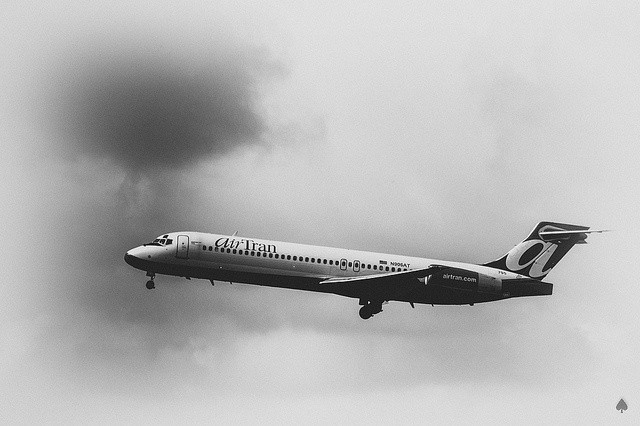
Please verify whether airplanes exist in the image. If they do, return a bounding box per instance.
[123,222,612,319]
